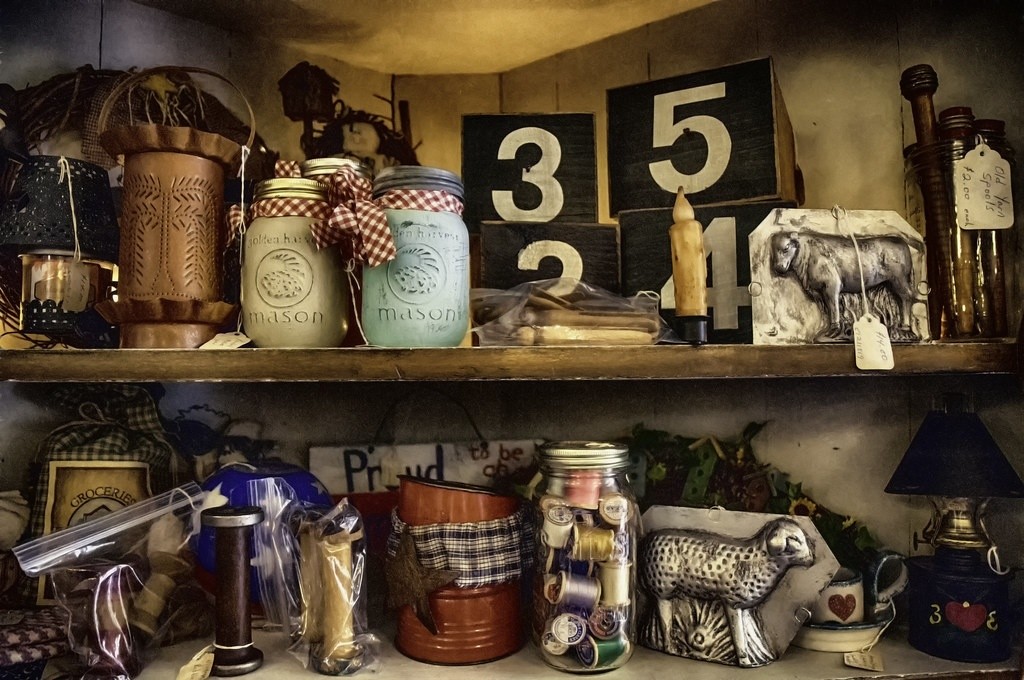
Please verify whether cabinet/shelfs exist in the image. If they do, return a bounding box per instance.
[0,339,1024,680]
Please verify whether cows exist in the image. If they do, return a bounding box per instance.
[769,227,919,339]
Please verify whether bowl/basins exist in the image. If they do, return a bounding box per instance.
[791,603,895,655]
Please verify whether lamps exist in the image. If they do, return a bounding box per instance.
[0,155,120,350]
[885,393,1023,663]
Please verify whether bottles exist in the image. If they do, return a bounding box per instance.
[357,165,472,348]
[297,156,372,348]
[238,178,354,349]
[531,438,640,674]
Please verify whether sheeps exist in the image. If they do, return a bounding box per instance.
[636,515,814,669]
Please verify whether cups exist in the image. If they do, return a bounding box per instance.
[811,568,865,627]
[904,135,1019,342]
[395,475,527,669]
